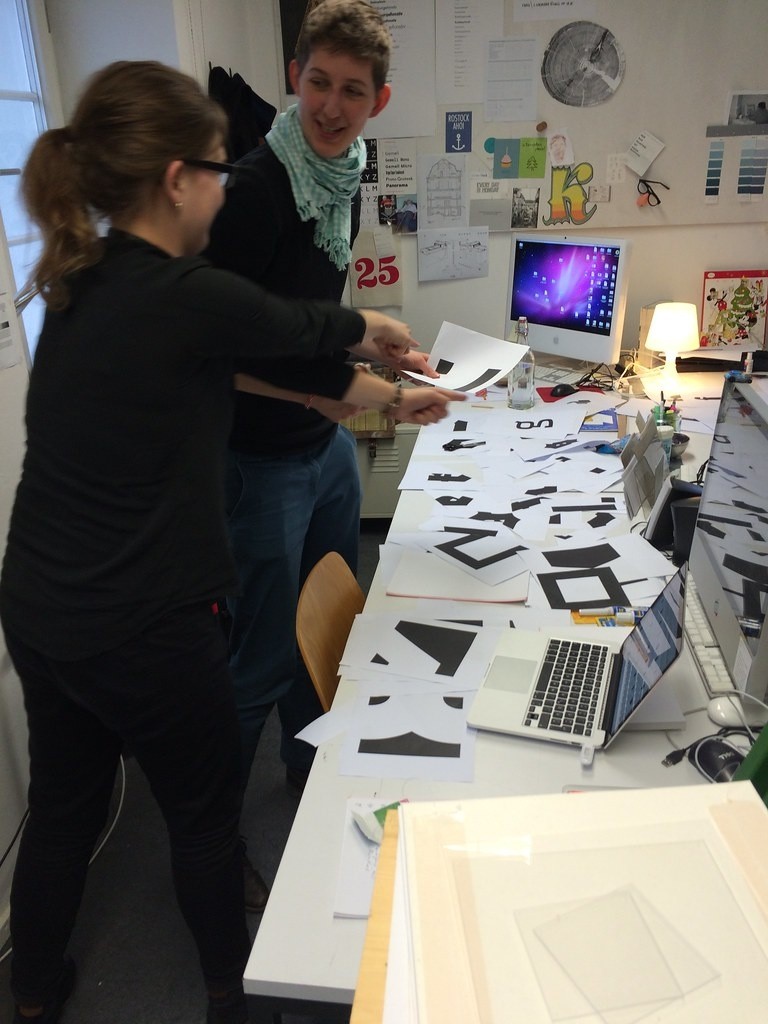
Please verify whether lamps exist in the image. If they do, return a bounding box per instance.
[645,301,700,391]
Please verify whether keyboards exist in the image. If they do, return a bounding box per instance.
[534,366,590,385]
[665,572,737,699]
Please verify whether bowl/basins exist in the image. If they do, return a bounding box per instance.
[671,432,690,457]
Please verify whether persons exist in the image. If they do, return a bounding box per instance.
[200,0,441,873]
[0,58,468,1024]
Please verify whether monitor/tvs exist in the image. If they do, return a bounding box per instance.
[505,232,631,375]
[687,375,768,702]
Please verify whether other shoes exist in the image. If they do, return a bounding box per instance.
[284,767,308,797]
[241,833,269,911]
[207,989,249,1023]
[14,953,76,1023]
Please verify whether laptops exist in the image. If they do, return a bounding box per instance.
[467,561,689,752]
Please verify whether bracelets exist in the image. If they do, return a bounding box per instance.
[304,392,317,410]
[381,384,403,419]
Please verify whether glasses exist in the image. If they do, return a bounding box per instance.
[163,153,244,191]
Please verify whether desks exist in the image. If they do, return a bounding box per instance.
[240,357,768,1009]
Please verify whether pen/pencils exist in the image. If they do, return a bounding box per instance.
[472,405,493,408]
[659,391,665,426]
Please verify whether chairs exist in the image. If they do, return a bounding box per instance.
[295,551,367,713]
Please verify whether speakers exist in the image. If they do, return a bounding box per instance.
[643,476,702,551]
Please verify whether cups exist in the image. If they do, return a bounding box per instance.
[656,426,674,467]
[647,408,680,432]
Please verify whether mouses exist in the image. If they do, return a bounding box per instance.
[708,696,768,728]
[550,384,579,397]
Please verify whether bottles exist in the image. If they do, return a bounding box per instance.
[744,352,754,372]
[507,316,535,410]
